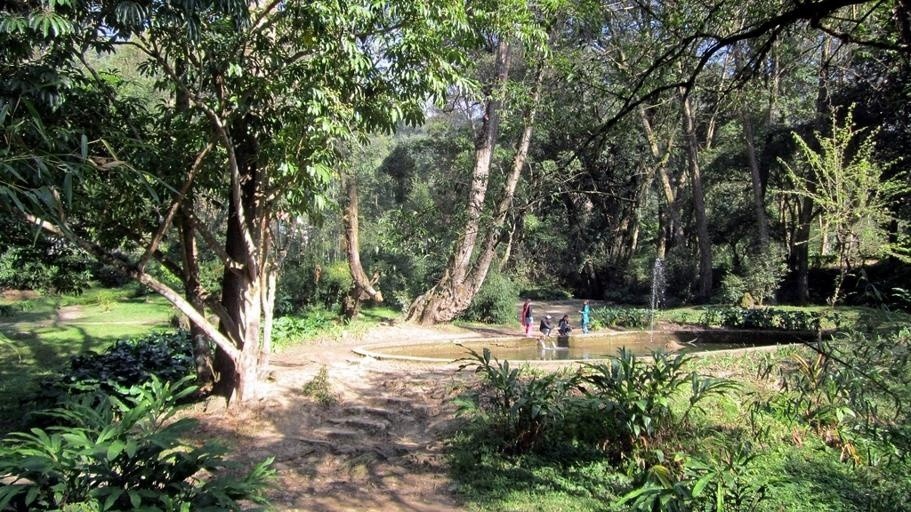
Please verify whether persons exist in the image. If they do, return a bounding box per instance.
[523,299,533,337]
[578,300,590,333]
[558,315,570,336]
[540,315,554,336]
